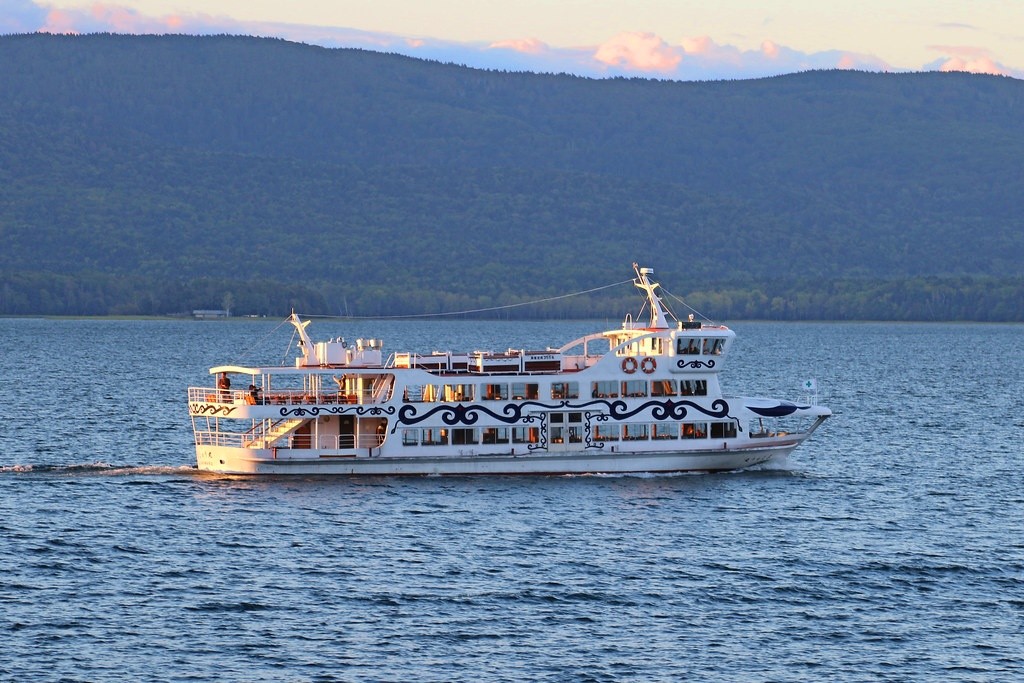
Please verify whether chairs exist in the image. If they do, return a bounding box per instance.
[205,393,348,404]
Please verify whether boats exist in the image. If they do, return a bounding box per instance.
[187,261,831,478]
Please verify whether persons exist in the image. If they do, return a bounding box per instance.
[248,385,271,405]
[678,339,694,354]
[703,339,708,351]
[218,372,232,402]
[376,418,387,446]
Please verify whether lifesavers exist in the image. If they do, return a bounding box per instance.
[641,357,657,374]
[621,357,638,374]
[342,341,347,348]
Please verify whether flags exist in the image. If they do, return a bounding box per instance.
[802,379,817,390]
